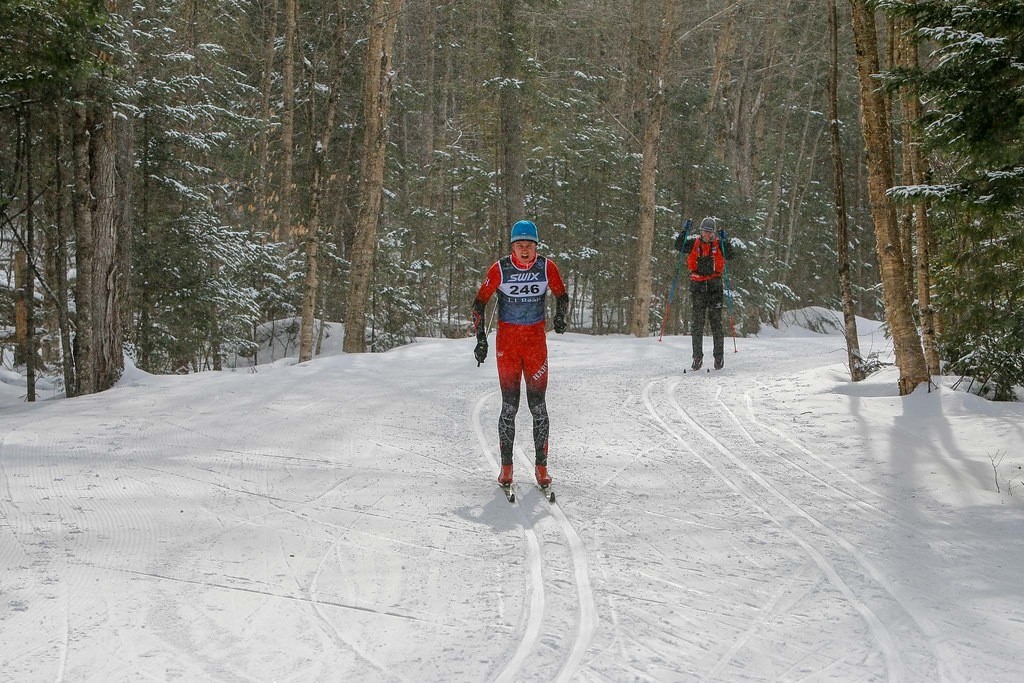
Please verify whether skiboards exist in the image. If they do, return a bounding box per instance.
[683,364,716,374]
[502,482,556,503]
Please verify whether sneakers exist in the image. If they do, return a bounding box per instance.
[498,464,513,485]
[692,357,703,370]
[714,355,724,369]
[534,465,552,485]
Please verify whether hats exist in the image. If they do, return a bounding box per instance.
[510,220,538,245]
[699,218,716,233]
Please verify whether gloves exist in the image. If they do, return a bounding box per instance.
[718,229,727,239]
[553,316,567,334]
[474,334,488,363]
[683,220,692,230]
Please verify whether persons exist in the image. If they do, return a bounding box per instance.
[675,217,736,371]
[472,220,570,485]
